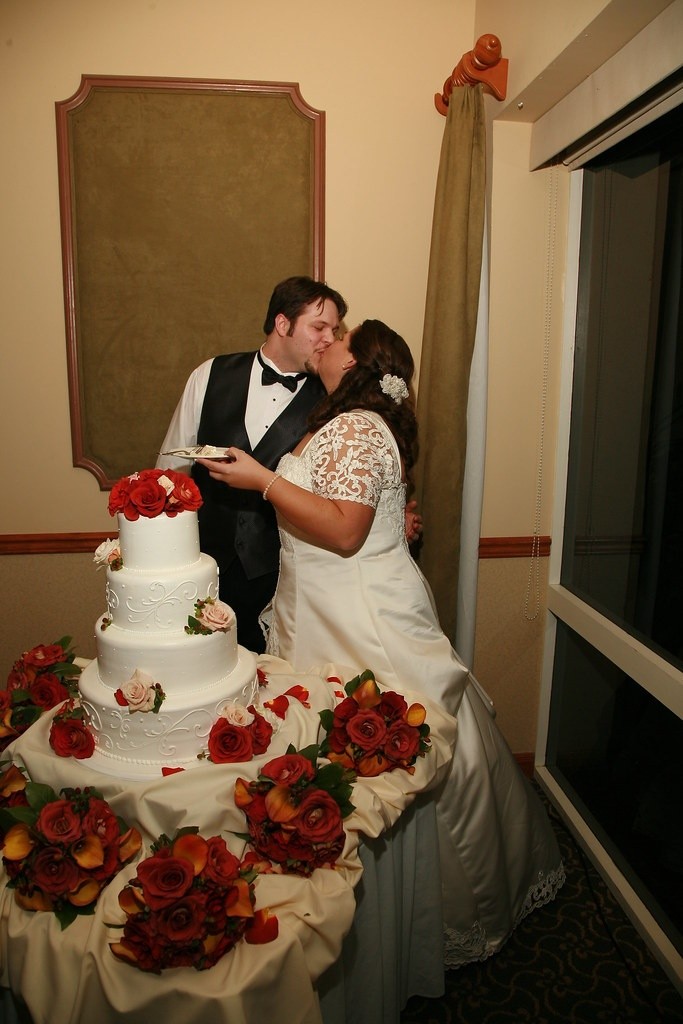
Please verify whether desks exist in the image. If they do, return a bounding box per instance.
[0,656,460,1024]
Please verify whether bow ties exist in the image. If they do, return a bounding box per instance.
[257,350,308,392]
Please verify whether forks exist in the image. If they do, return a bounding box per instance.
[154,443,203,456]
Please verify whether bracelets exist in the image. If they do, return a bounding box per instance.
[263,473,281,499]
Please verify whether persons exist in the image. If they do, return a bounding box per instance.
[151,275,423,654]
[195,319,566,968]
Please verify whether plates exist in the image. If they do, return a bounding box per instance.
[170,446,244,461]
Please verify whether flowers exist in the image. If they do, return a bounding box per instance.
[225,742,357,877]
[378,374,409,406]
[317,668,434,777]
[107,467,204,521]
[93,537,121,571]
[119,668,167,713]
[102,825,258,976]
[208,701,273,763]
[1,760,143,931]
[0,634,95,759]
[183,596,237,636]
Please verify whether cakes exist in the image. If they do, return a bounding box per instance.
[49,468,274,766]
